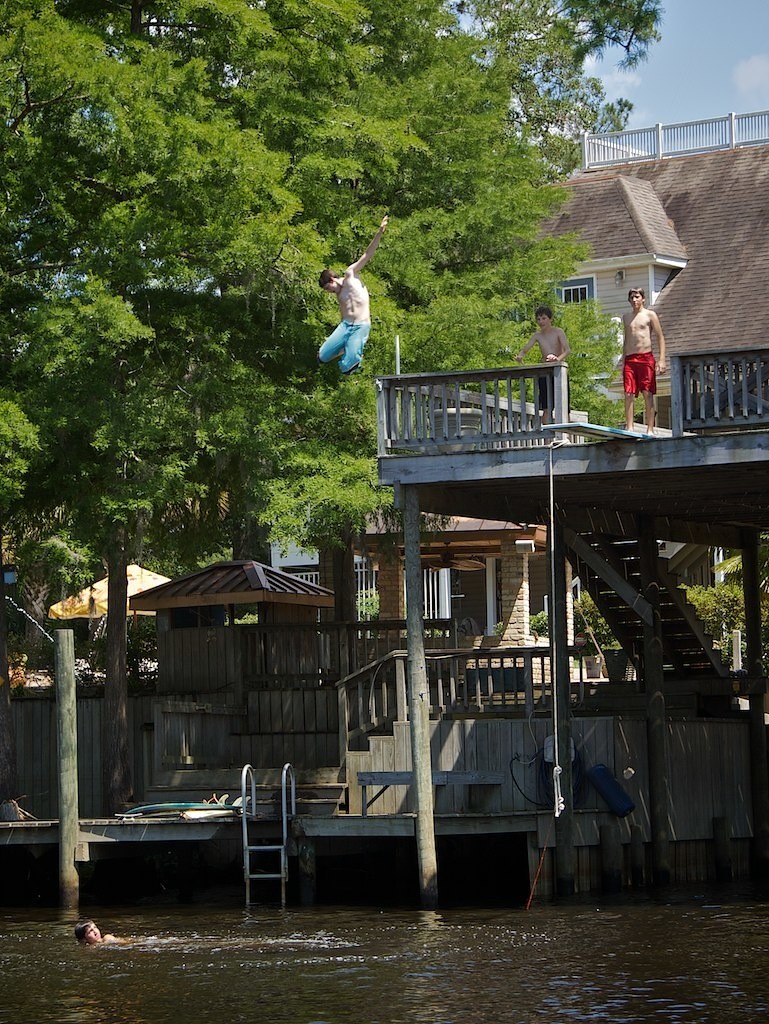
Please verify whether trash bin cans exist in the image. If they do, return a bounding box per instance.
[584,656,601,678]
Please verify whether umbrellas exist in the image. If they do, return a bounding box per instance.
[48,562,172,629]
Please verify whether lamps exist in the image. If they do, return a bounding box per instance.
[614,269,625,288]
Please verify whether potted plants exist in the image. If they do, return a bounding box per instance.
[573,591,628,681]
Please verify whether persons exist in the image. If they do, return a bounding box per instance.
[75,920,115,944]
[514,307,571,444]
[623,288,665,436]
[318,215,390,375]
